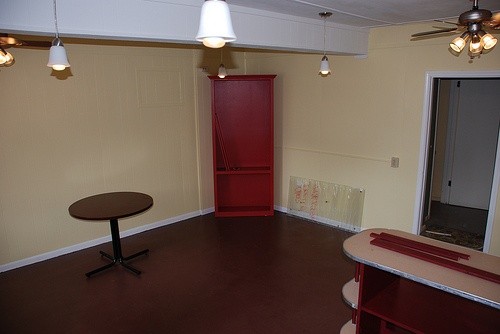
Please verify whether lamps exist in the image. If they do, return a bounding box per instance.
[449,24,498,53]
[47,0,70,70]
[0,33,19,65]
[196,0,237,48]
[217,47,228,78]
[318,12,334,75]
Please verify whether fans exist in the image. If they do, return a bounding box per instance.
[411,0,499,38]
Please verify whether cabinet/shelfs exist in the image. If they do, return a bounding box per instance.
[338,229,500,334]
[208,74,278,216]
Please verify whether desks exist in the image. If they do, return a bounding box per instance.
[69,191,153,279]
